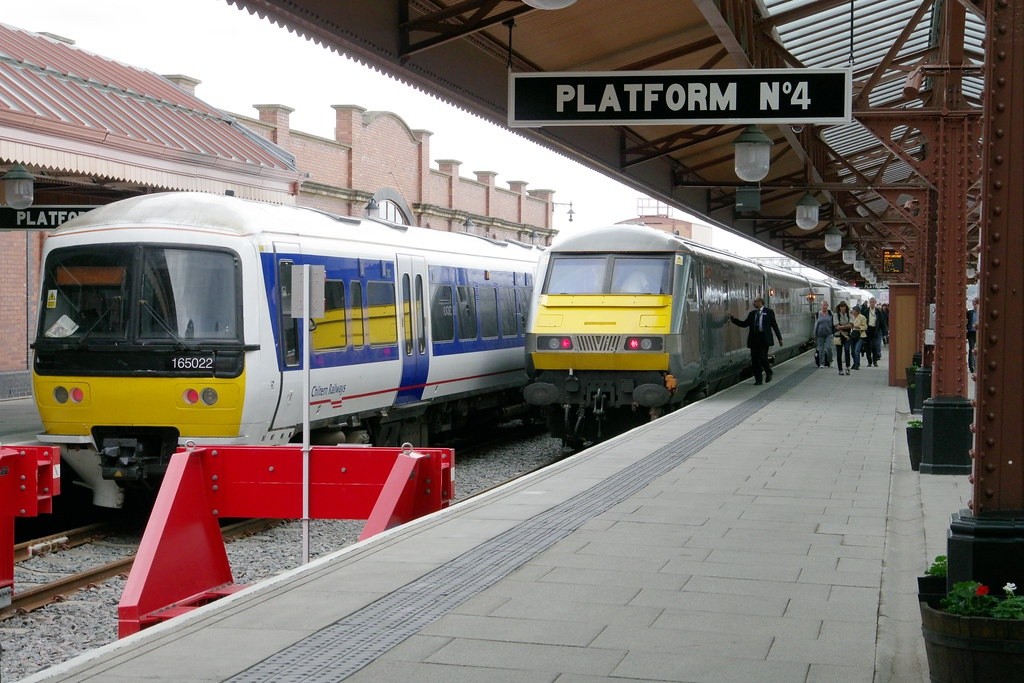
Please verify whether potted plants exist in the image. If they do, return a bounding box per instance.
[907,420,923,472]
[906,366,919,413]
[918,556,948,595]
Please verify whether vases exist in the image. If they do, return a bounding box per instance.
[920,600,1024,683]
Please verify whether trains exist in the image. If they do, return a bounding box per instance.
[523,222,869,449]
[30,191,548,521]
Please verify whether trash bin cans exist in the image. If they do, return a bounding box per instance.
[913,352,922,367]
[919,394,974,475]
[914,367,932,412]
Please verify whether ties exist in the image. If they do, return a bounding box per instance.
[754,311,761,337]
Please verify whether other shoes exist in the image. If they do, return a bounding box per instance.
[851,364,859,370]
[765,371,773,383]
[867,363,871,367]
[839,371,844,375]
[820,365,824,369]
[845,369,850,375]
[873,362,878,367]
[754,382,762,385]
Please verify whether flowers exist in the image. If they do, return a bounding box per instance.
[941,581,1024,620]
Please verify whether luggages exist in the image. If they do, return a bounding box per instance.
[814,339,830,368]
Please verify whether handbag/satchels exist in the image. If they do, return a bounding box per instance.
[851,330,860,343]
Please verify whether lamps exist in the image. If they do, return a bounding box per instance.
[365,198,379,217]
[853,254,877,284]
[824,222,843,254]
[732,125,774,182]
[0,164,36,210]
[903,66,926,99]
[528,230,539,245]
[840,243,857,264]
[904,200,919,213]
[794,190,821,230]
[552,202,575,222]
[460,217,474,235]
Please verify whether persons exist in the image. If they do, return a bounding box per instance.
[966,298,978,372]
[730,297,783,385]
[815,297,889,375]
[706,311,731,357]
